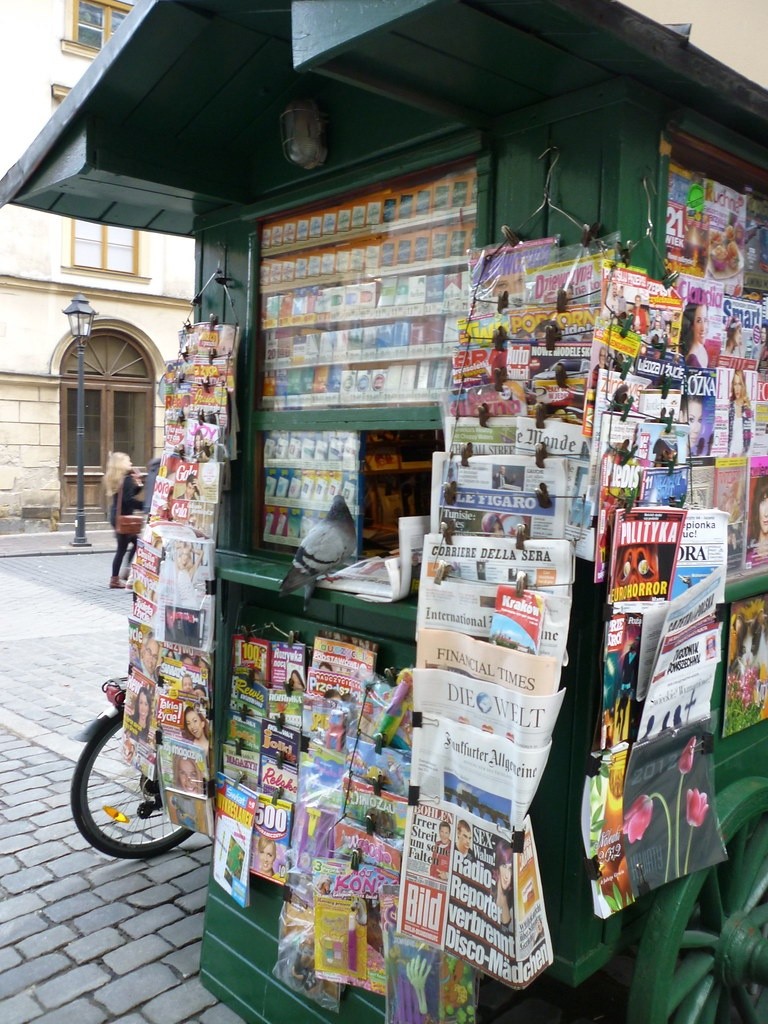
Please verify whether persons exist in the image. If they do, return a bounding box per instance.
[145,458,161,524]
[481,295,768,569]
[129,475,513,924]
[101,452,144,588]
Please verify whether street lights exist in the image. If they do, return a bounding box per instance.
[61,293,99,548]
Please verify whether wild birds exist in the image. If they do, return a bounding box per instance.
[278,495,356,611]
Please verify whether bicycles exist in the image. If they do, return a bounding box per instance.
[69,678,195,860]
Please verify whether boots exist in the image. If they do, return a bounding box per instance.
[110,577,126,588]
[124,569,132,580]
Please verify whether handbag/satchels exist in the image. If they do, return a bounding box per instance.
[116,515,144,534]
